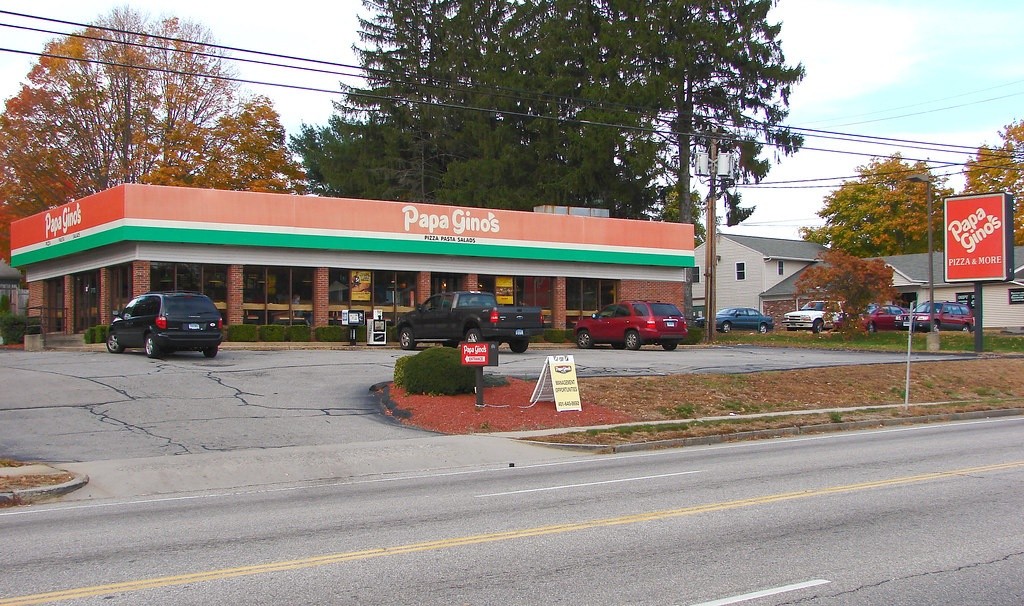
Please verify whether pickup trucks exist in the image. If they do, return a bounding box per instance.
[395,289,544,353]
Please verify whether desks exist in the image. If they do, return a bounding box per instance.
[274,316,305,321]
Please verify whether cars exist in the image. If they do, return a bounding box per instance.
[833,304,909,332]
[694,307,775,334]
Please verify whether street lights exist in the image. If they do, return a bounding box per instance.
[906,172,940,354]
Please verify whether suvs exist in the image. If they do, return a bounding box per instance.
[573,299,689,351]
[894,299,977,332]
[782,300,847,332]
[106,289,224,359]
